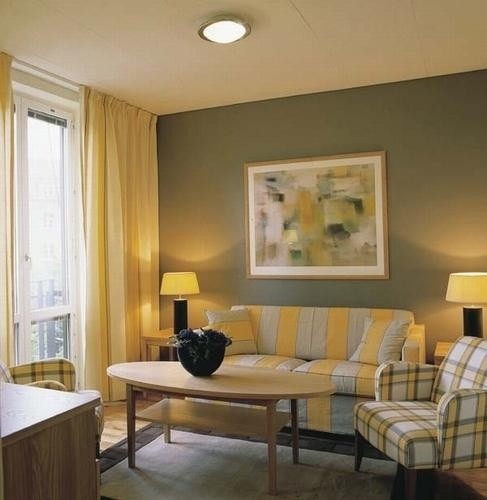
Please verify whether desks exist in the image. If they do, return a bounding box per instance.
[139,326,179,400]
[431,341,457,367]
[105,359,337,495]
[0,379,106,500]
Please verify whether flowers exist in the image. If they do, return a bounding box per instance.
[162,324,234,366]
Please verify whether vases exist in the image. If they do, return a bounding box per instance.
[175,340,228,378]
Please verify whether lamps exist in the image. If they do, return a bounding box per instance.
[194,10,252,45]
[156,268,201,336]
[443,271,486,338]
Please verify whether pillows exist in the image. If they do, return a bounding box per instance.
[347,315,413,368]
[201,304,259,357]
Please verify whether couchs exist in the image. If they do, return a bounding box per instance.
[180,302,428,446]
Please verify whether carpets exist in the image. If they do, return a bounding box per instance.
[95,418,413,500]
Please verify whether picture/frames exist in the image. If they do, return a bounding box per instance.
[241,148,393,283]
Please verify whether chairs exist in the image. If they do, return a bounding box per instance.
[0,355,105,462]
[351,332,486,500]
